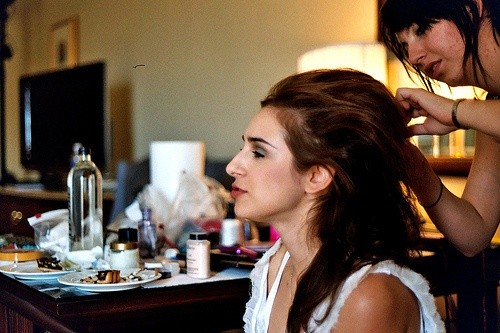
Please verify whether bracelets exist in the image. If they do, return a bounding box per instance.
[418,175,443,208]
[452,98,470,130]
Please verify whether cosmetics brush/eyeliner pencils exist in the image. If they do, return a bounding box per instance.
[164,248,219,264]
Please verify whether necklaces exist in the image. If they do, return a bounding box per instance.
[289,264,294,301]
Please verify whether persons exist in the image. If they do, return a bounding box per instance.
[378,0,500,258]
[226,67,446,333]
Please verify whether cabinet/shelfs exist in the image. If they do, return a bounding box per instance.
[0,183,121,238]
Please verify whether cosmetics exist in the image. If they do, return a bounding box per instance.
[185,232,211,278]
[110,228,140,270]
[222,203,258,258]
[67,146,105,252]
[138,208,156,259]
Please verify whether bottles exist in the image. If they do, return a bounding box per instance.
[138,208,157,262]
[186,232,211,279]
[220,201,243,246]
[66,147,102,253]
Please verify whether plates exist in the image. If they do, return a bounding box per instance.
[210,246,273,268]
[0,259,163,293]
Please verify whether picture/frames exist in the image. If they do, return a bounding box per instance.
[47,15,80,70]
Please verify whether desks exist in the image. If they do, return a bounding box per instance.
[0,234,436,332]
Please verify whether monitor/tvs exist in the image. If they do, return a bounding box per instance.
[19,60,111,190]
